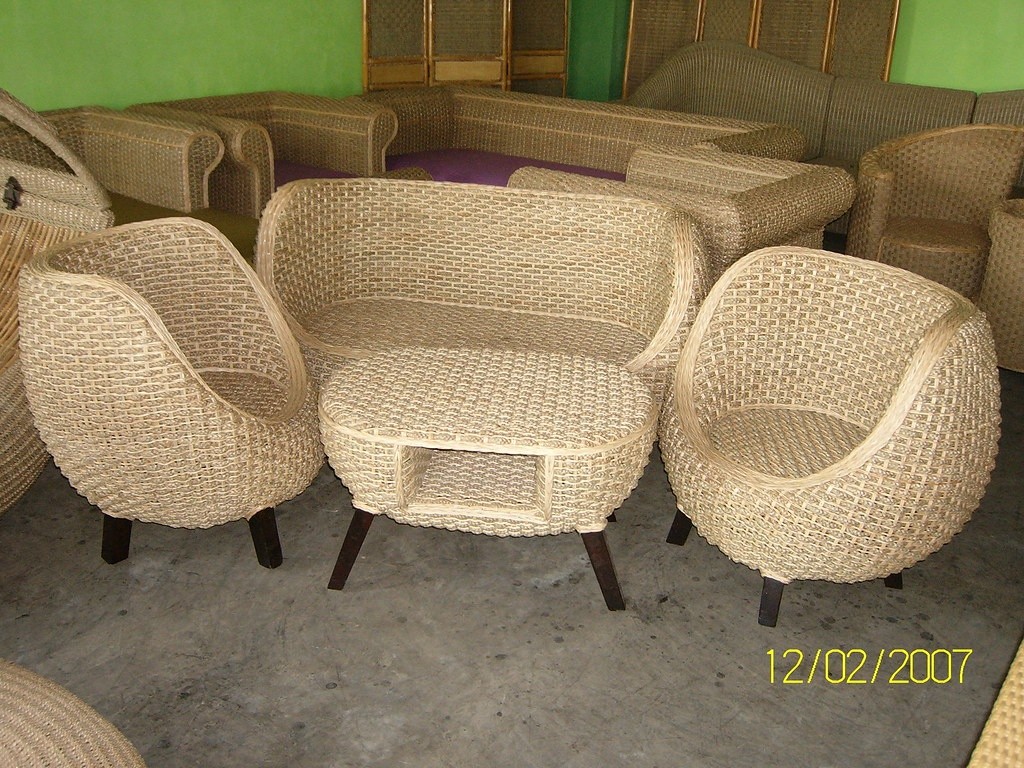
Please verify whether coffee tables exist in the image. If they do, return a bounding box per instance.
[317,349,657,612]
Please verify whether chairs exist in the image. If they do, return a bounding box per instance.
[656,244,1003,628]
[845,123,1024,294]
[126,91,400,220]
[0,105,258,258]
[19,217,324,568]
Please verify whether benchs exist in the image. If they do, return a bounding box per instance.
[625,41,1024,237]
[345,85,804,188]
[258,177,712,523]
